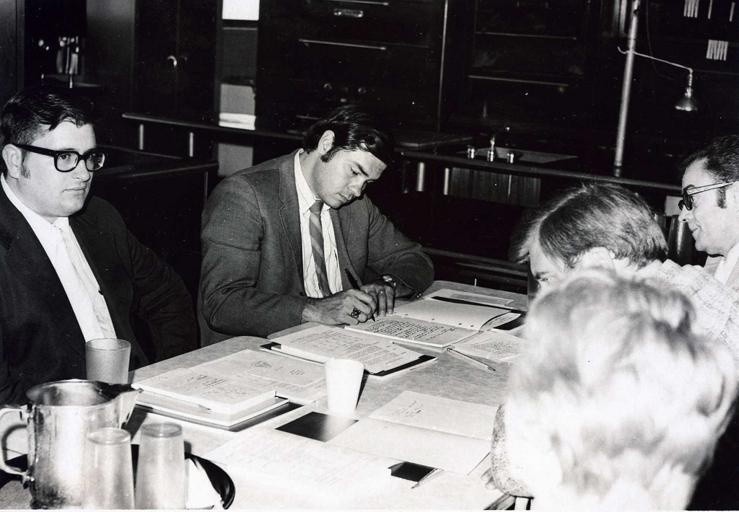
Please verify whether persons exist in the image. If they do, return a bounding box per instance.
[0,87,196,407]
[197,104,436,347]
[492,180,738,499]
[504,266,738,510]
[677,137,739,289]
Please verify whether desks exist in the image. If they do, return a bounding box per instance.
[1,280,530,510]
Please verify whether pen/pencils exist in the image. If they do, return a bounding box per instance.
[344,268,375,323]
[447,348,496,372]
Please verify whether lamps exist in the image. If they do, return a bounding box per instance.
[617,46,699,111]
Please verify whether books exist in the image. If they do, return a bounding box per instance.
[132,294,527,477]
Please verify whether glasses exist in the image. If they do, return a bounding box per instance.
[14,143,106,172]
[679,182,733,210]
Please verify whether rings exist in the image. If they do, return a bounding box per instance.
[351,308,361,319]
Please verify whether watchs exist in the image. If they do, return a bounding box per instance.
[379,275,398,289]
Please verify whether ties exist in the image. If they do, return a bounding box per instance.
[309,200,333,298]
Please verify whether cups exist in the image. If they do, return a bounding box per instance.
[0,337,185,509]
[325,358,365,414]
